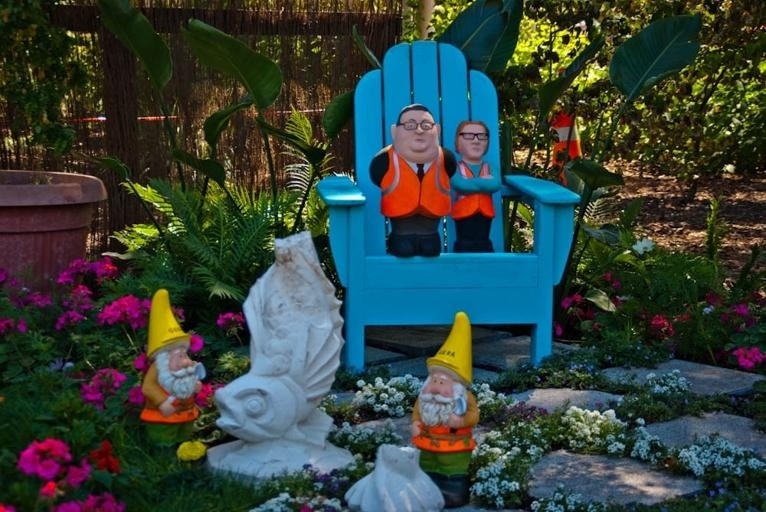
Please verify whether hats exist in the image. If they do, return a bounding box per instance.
[145,287,192,358]
[425,310,475,385]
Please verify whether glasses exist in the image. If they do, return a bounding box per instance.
[397,121,437,131]
[457,132,488,142]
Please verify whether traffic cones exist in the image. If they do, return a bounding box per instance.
[553,67,583,186]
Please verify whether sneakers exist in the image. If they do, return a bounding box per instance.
[440,475,472,504]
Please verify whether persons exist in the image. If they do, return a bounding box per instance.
[453,120,495,253]
[408,311,480,506]
[371,102,455,259]
[142,287,205,452]
